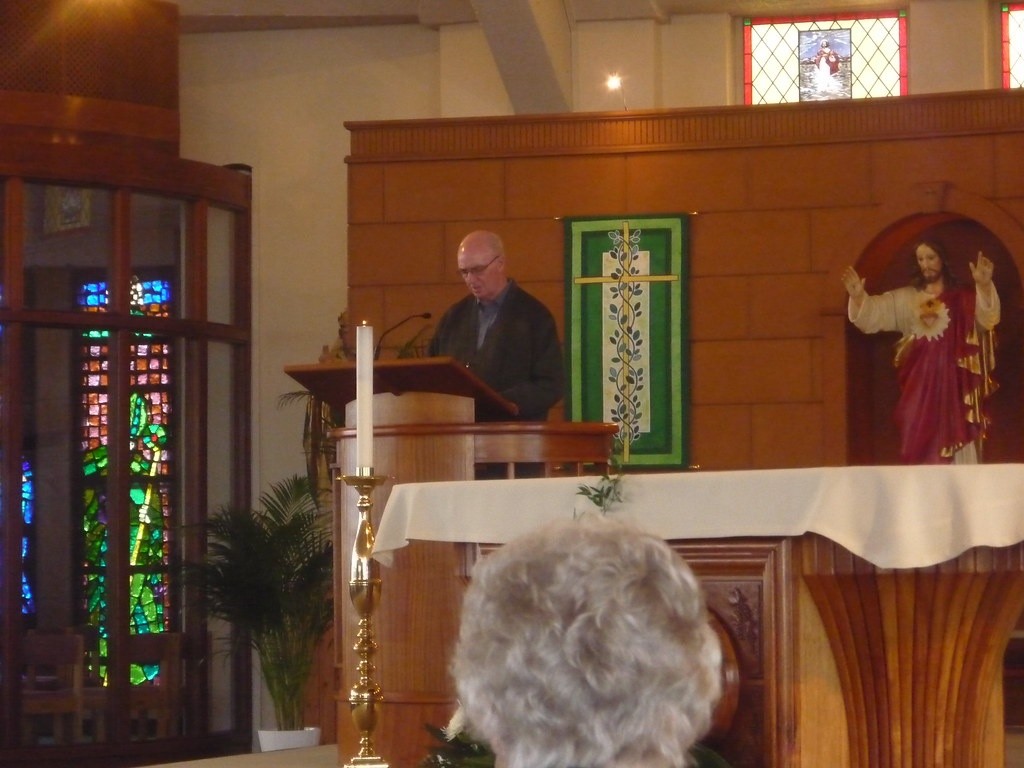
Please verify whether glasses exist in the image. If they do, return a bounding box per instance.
[456,256,498,275]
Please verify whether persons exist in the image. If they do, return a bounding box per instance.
[839,238,1003,465]
[445,510,724,767]
[425,229,565,483]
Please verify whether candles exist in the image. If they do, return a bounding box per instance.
[356,319,373,475]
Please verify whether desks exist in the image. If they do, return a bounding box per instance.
[370,464,1024,766]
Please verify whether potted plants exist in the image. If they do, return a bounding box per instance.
[180,474,342,754]
